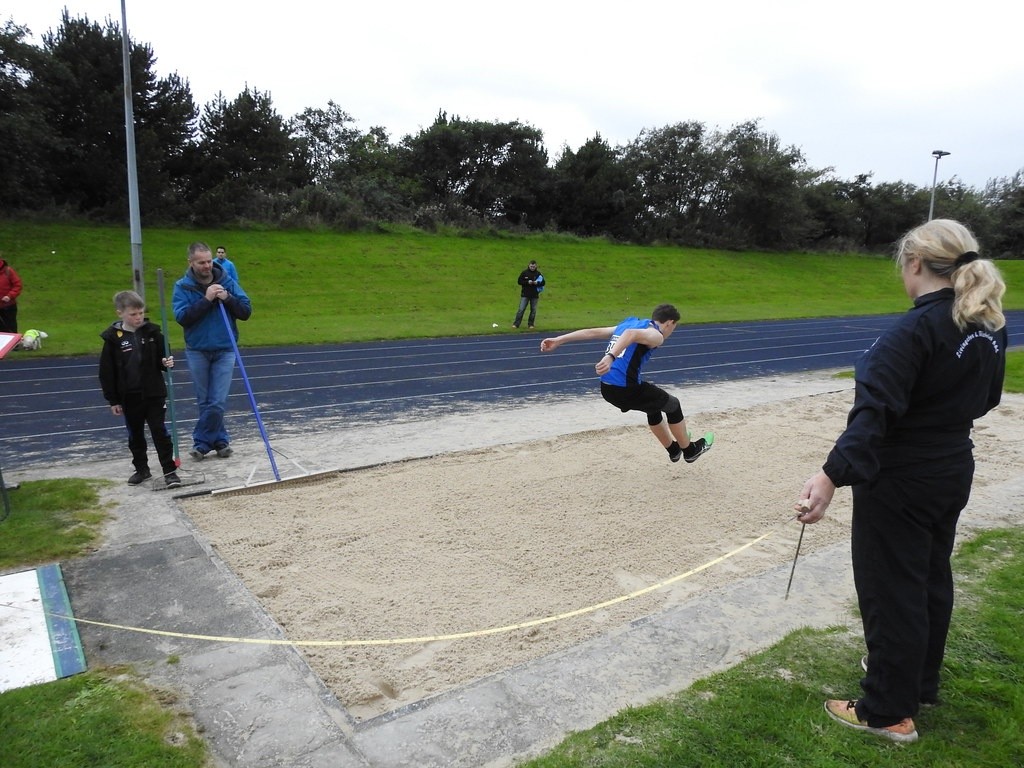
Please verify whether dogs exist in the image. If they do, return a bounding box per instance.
[20,329,49,351]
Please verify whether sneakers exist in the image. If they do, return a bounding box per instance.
[217,445,234,457]
[163,471,181,488]
[669,432,692,462]
[188,449,203,462]
[684,432,714,463]
[824,698,919,744]
[860,654,937,708]
[127,466,153,485]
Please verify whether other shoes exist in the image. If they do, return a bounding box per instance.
[528,326,534,329]
[512,325,517,329]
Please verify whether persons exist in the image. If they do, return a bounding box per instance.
[512,260,545,329]
[172,241,252,460]
[541,304,713,464]
[99,290,181,488]
[795,218,1009,740]
[0,258,22,351]
[212,246,239,343]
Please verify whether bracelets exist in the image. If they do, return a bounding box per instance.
[607,353,615,362]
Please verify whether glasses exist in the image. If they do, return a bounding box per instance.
[217,252,224,254]
